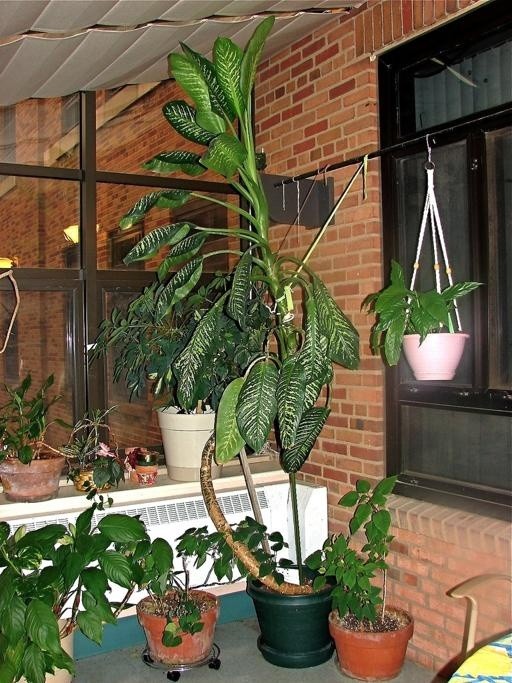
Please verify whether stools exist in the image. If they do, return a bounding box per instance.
[431,573,512,682]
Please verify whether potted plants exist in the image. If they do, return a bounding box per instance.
[88,16,359,669]
[2,368,161,500]
[360,254,483,385]
[302,472,417,683]
[2,504,168,683]
